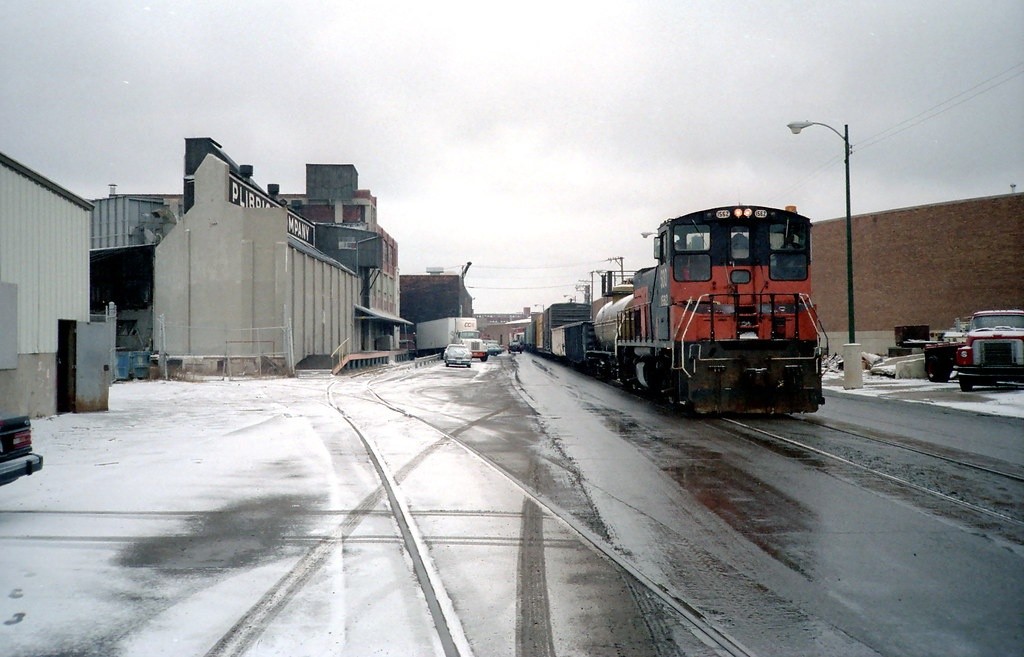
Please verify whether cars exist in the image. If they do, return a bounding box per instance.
[483,340,506,356]
[508,340,522,354]
[447,347,472,368]
[444,344,465,363]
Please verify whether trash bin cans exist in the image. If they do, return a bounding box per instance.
[133,351,150,378]
[115,351,131,379]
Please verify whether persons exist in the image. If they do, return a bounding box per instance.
[780,234,801,249]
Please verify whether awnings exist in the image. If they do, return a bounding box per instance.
[354,305,414,326]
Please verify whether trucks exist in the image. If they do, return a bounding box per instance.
[416,317,489,362]
[889,309,1024,392]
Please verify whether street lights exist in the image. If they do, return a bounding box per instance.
[535,303,544,311]
[563,294,577,302]
[787,120,863,390]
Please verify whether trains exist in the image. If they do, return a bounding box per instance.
[509,205,830,419]
